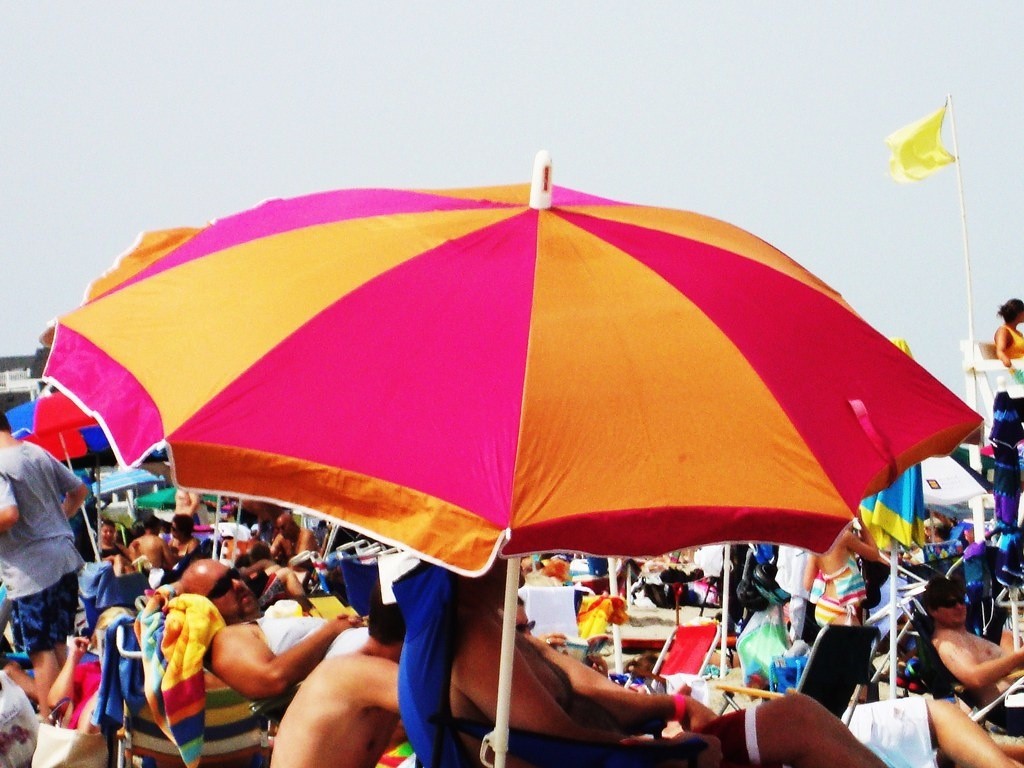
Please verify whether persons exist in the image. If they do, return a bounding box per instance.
[0,298,1024,768]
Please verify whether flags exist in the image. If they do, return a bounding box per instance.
[883,99,956,185]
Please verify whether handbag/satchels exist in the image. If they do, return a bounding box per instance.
[1,670,111,767]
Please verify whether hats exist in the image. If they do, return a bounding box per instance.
[923,517,943,528]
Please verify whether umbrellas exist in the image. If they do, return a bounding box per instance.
[4,149,985,768]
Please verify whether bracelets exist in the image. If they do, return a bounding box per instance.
[668,693,686,723]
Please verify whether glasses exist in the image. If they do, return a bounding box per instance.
[937,596,965,608]
[207,568,241,598]
[516,620,536,634]
[275,525,287,531]
[171,526,178,533]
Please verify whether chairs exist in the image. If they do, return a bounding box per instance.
[81,539,1024,768]
